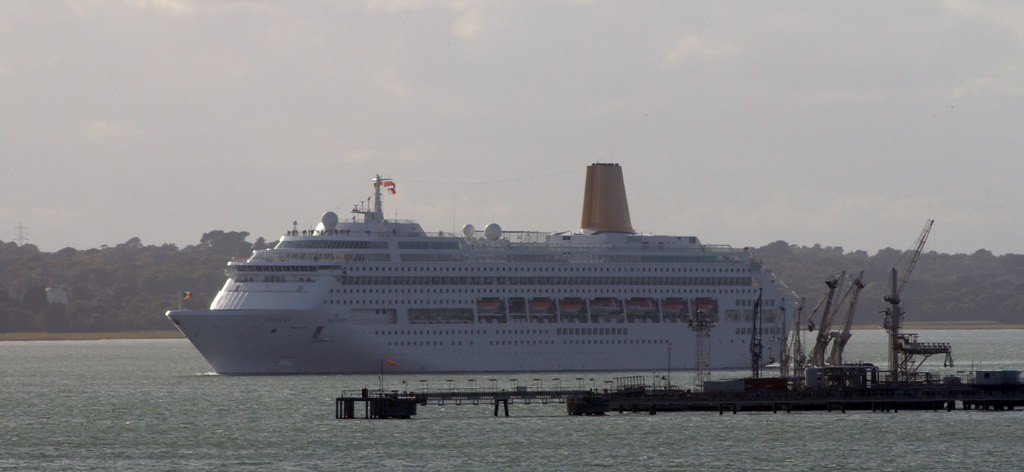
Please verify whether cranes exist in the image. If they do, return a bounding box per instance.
[882,217,957,374]
[748,266,867,388]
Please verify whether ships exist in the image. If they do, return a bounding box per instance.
[166,160,794,375]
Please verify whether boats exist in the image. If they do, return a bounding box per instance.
[588,298,622,316]
[478,300,502,311]
[557,301,584,313]
[661,300,689,314]
[529,300,553,312]
[693,300,716,314]
[625,297,658,315]
